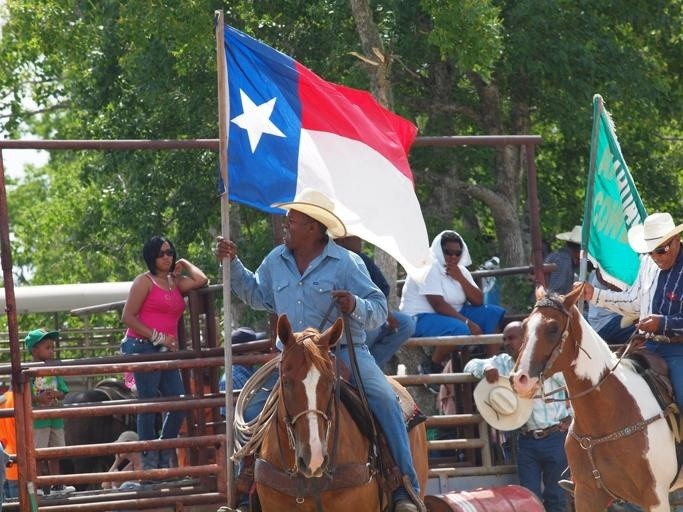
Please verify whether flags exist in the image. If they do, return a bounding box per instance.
[221,18,432,287]
[584,92,646,295]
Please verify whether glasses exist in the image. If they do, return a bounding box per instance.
[647,237,675,254]
[155,248,175,257]
[441,248,462,257]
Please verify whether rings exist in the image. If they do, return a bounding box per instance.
[171,341,175,346]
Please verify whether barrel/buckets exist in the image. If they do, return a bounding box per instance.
[424,483,543,511]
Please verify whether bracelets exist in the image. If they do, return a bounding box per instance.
[148,327,165,347]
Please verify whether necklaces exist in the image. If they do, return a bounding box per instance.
[154,271,172,291]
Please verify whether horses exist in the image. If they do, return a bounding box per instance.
[250,311,430,512]
[506,278,682,512]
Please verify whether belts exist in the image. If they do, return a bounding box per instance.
[645,334,683,344]
[514,424,563,440]
[328,343,363,350]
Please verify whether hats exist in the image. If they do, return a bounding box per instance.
[230,327,269,344]
[626,212,683,254]
[472,375,534,432]
[554,225,583,244]
[270,189,347,238]
[24,327,59,353]
[113,430,140,443]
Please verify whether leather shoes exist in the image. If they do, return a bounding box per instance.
[217,505,248,512]
[558,479,575,494]
[395,499,417,512]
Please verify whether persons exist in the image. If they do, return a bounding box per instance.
[331,235,416,372]
[462,319,576,511]
[215,190,426,511]
[116,232,209,485]
[99,429,140,489]
[397,228,507,397]
[0,373,18,497]
[217,325,270,418]
[23,325,76,498]
[580,211,682,417]
[531,221,582,298]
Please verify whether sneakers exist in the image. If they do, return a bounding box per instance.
[141,476,183,490]
[35,483,77,498]
[417,359,443,395]
